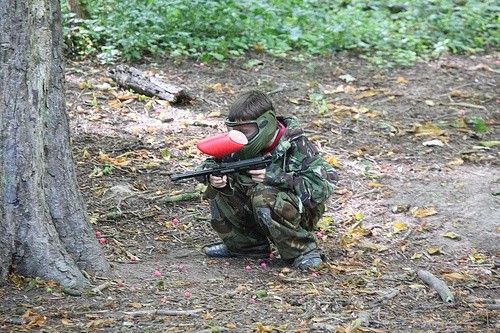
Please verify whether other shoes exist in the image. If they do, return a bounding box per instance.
[201,240,271,259]
[295,251,323,270]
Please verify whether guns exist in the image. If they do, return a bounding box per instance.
[170,130,272,189]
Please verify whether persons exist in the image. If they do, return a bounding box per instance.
[195,91,339,273]
